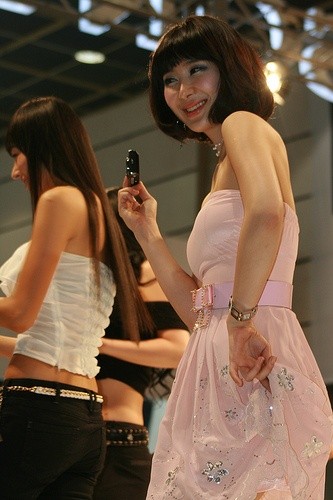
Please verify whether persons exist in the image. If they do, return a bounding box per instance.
[0,186,194,500]
[0,97,154,500]
[117,17,333,500]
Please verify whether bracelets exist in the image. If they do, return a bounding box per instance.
[228,294,259,321]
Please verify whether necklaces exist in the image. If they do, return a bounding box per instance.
[212,136,225,156]
